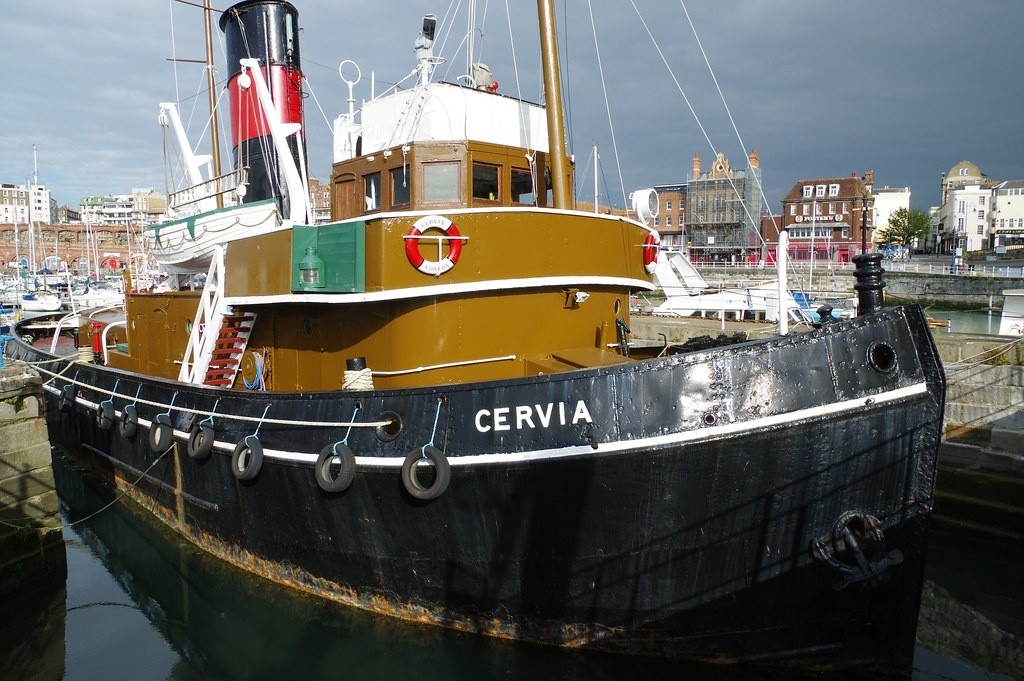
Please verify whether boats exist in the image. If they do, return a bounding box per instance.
[0,0,1023,681]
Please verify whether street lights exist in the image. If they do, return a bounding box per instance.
[860,194,868,254]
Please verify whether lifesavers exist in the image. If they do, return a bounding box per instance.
[642,228,661,276]
[405,218,461,276]
